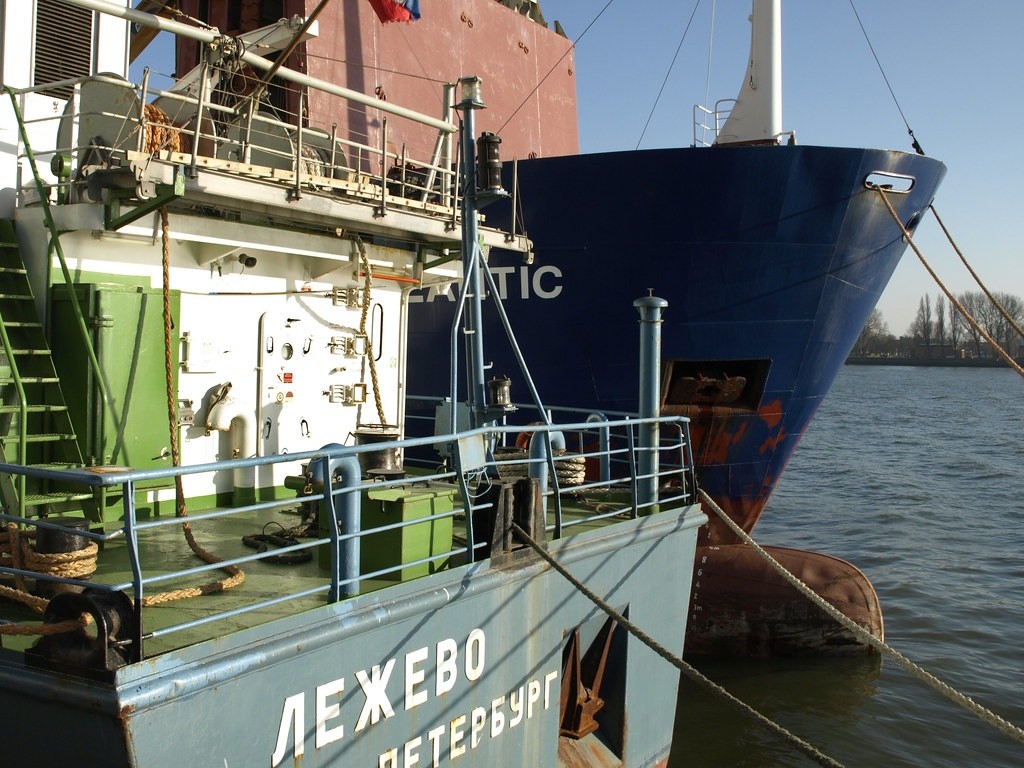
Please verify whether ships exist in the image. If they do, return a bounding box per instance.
[0,1,952,768]
